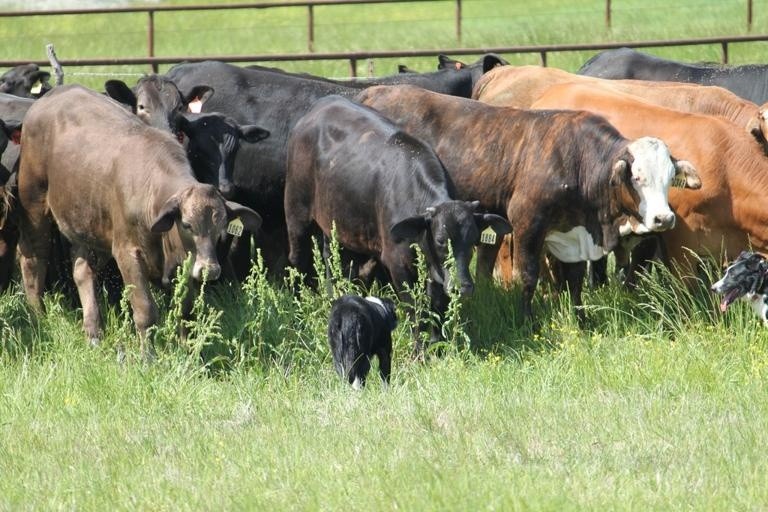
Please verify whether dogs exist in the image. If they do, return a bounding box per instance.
[327,293,398,394]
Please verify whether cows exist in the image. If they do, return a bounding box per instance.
[0,46,768,370]
